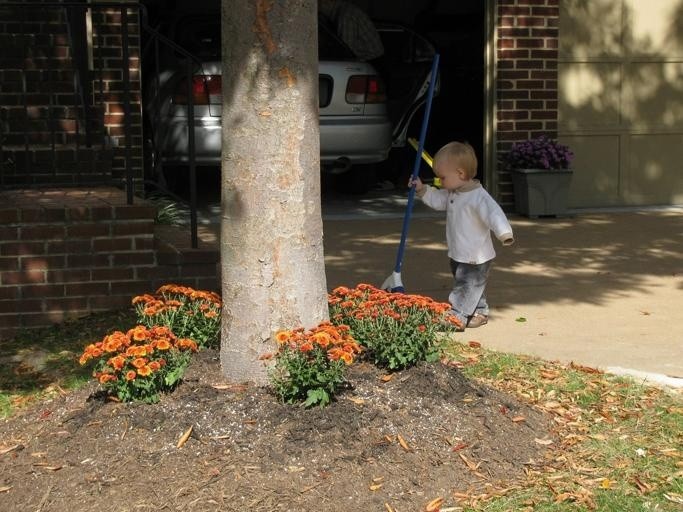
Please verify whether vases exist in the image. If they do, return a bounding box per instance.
[510,165,575,219]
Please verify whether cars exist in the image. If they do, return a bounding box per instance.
[141,13,392,196]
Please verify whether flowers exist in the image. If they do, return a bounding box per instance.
[505,134,575,170]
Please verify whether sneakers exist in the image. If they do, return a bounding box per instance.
[437,317,464,332]
[466,315,489,328]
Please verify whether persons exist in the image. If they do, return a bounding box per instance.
[408,143,516,330]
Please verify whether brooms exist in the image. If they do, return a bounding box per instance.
[378,52,440,295]
